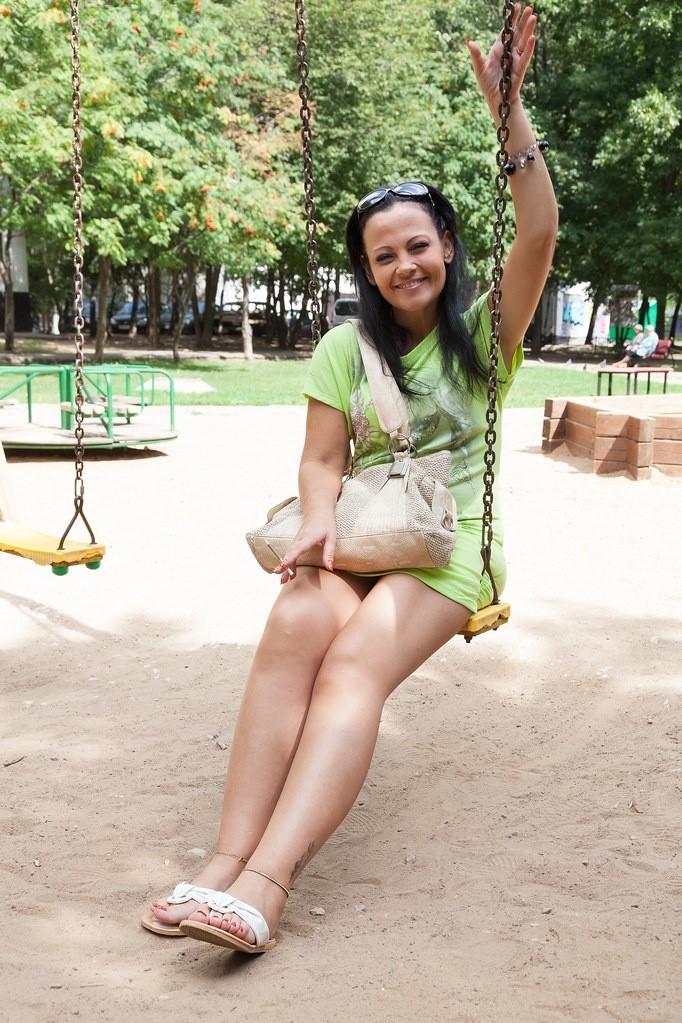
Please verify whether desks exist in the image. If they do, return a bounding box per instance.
[598,367,670,395]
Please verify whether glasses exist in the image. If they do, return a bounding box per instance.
[357,181,436,220]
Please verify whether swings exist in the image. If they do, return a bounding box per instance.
[294,0,516,642]
[0,0,109,579]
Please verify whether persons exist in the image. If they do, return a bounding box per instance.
[140,1,558,953]
[612,324,659,368]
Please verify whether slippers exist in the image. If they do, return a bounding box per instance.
[141,881,224,936]
[179,892,276,954]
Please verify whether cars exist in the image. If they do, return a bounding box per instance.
[110,301,182,333]
[160,301,222,335]
[258,310,328,336]
[58,300,123,334]
[331,299,362,328]
[219,301,288,337]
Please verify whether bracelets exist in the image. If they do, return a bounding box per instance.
[502,140,549,177]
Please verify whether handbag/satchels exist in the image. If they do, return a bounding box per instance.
[245,319,457,574]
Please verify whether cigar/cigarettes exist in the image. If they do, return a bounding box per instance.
[264,540,294,576]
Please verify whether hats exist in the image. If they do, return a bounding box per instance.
[646,326,654,332]
[634,324,643,330]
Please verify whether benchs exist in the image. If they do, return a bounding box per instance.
[618,339,675,367]
